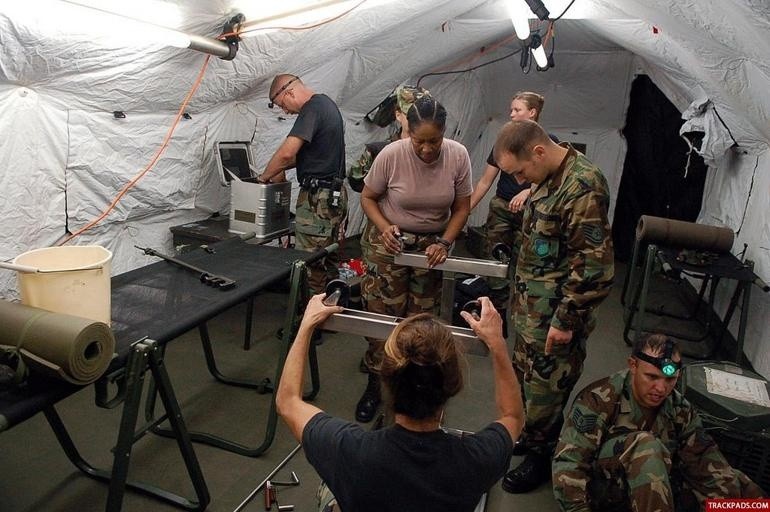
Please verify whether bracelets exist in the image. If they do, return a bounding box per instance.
[256,172,266,184]
[434,236,452,250]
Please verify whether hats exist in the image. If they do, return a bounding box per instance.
[398,85,433,114]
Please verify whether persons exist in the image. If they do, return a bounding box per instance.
[548,332,770,510]
[355,95,476,423]
[490,113,617,495]
[466,89,559,339]
[255,72,348,349]
[348,82,433,374]
[274,288,530,511]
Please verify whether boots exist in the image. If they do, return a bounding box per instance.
[499,435,552,494]
[356,373,381,424]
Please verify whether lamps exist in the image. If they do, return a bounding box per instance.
[169,15,246,60]
[528,35,550,71]
[506,0,531,41]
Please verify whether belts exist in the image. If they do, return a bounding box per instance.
[401,231,426,246]
[318,181,331,190]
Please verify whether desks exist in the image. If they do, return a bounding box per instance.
[0,239,339,512]
[620,236,770,370]
[169,215,298,349]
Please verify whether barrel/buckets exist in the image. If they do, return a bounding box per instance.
[0,243,114,327]
